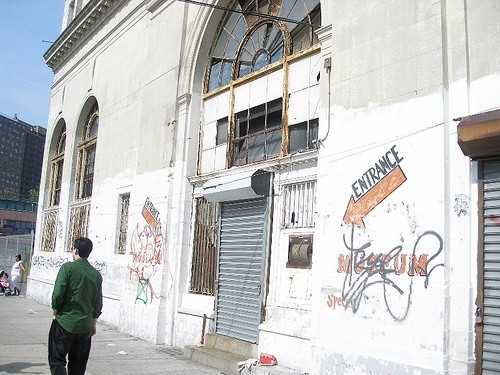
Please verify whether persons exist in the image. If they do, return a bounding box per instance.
[1,255,26,297]
[47,238,103,375]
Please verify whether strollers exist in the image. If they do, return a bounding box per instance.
[0,270,13,296]
